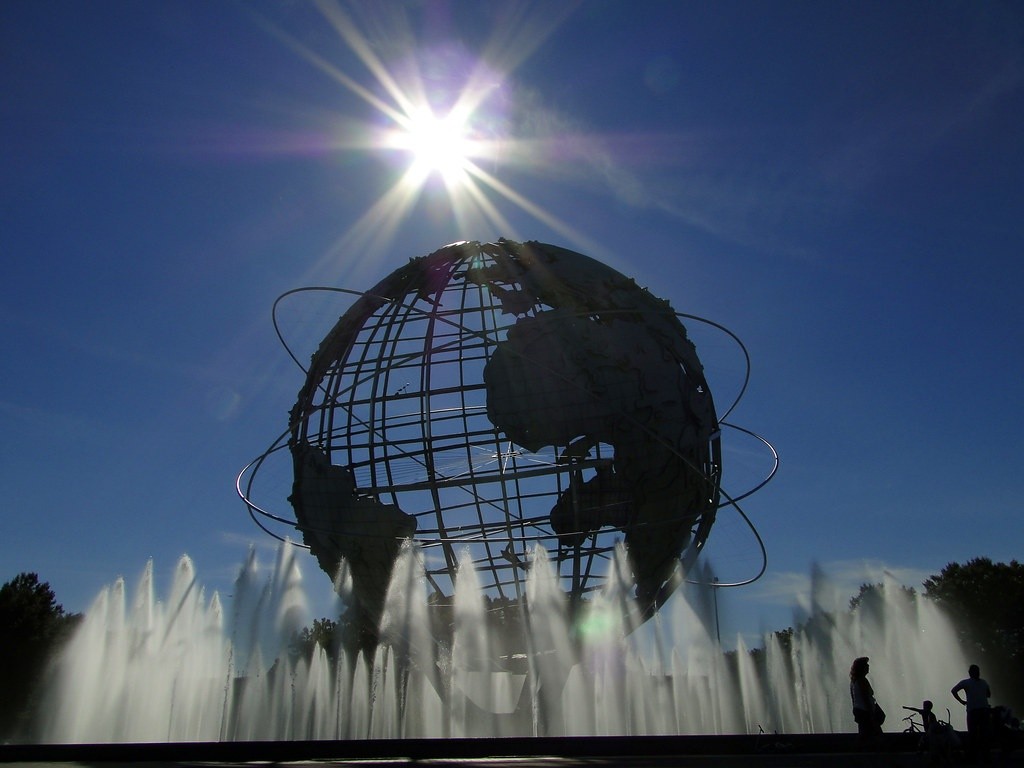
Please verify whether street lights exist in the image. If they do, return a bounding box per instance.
[711,576,721,644]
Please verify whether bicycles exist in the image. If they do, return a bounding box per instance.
[902,713,949,740]
[752,725,793,754]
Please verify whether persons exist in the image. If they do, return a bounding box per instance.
[849,657,883,753]
[951,665,993,768]
[903,701,940,755]
